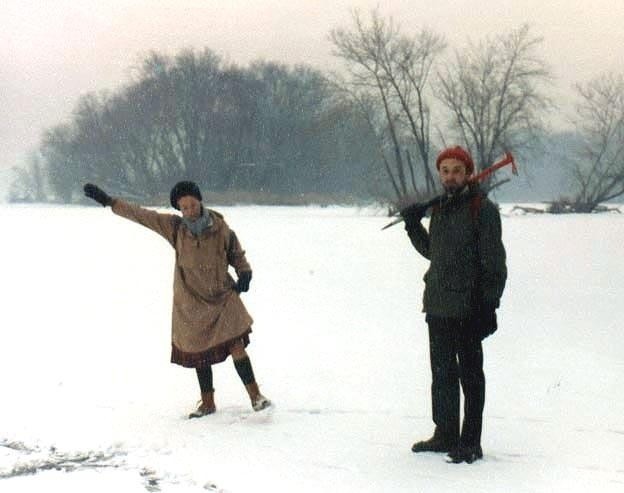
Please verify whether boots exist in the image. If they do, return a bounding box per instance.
[245,381,272,412]
[188,389,216,418]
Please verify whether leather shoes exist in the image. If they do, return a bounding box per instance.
[450,441,482,463]
[412,434,462,454]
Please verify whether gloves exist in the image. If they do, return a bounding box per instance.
[400,202,425,229]
[82,183,111,208]
[236,273,252,293]
[470,308,498,341]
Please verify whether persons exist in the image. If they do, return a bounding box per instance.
[82,177,270,417]
[401,144,507,463]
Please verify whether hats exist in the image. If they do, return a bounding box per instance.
[436,144,475,177]
[169,180,202,211]
